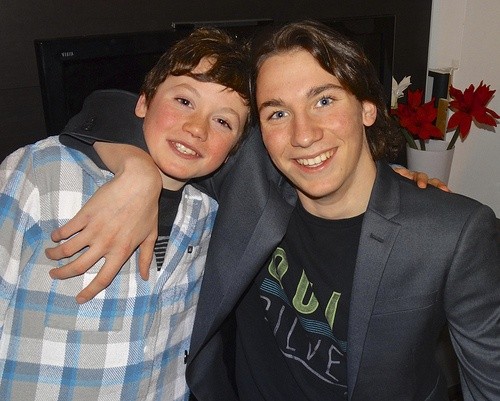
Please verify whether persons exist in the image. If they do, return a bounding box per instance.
[42,18,500,401]
[0,25,453,401]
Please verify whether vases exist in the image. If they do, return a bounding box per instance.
[406,138,455,186]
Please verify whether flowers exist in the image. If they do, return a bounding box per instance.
[390,74,500,150]
